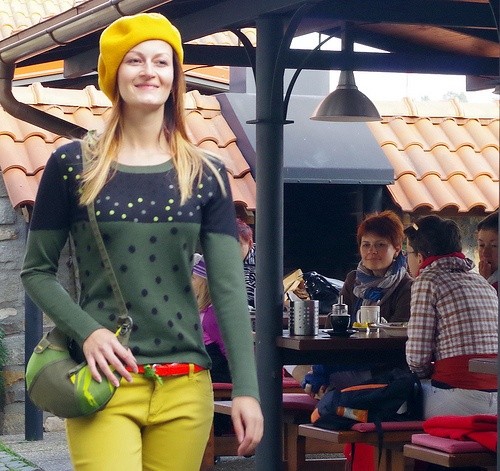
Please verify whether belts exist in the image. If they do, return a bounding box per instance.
[122,360,209,379]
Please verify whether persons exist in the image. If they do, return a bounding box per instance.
[19,12,268,471]
[188,206,500,419]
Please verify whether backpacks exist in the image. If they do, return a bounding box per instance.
[310,370,417,432]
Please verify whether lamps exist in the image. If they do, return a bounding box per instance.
[310,25,385,123]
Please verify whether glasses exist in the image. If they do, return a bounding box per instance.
[402,246,419,259]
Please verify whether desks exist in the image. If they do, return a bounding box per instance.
[250,312,328,329]
[255,329,408,471]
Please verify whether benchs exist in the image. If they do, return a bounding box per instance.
[202,376,500,471]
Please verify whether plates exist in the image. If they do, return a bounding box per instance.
[322,330,359,337]
[352,326,378,331]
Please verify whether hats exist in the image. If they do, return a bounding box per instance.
[96,12,186,102]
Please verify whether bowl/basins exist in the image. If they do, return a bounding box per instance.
[371,322,407,336]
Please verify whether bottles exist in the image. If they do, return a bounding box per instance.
[337,406,367,423]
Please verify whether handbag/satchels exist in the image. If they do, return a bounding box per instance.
[24,313,133,421]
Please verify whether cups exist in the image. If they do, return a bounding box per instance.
[356,306,380,323]
[328,312,351,332]
